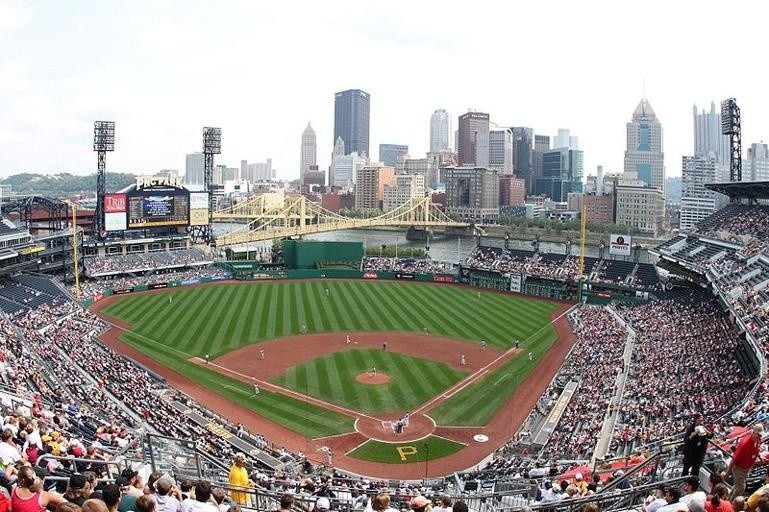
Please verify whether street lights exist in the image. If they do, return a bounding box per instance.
[92,116,116,246]
[200,126,223,244]
[718,95,741,206]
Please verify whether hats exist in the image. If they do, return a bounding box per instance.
[754,422,766,435]
[121,467,138,480]
[315,496,330,511]
[158,475,176,492]
[114,476,132,488]
[70,474,85,489]
[614,469,624,476]
[576,472,583,480]
[411,496,431,509]
[40,434,53,443]
